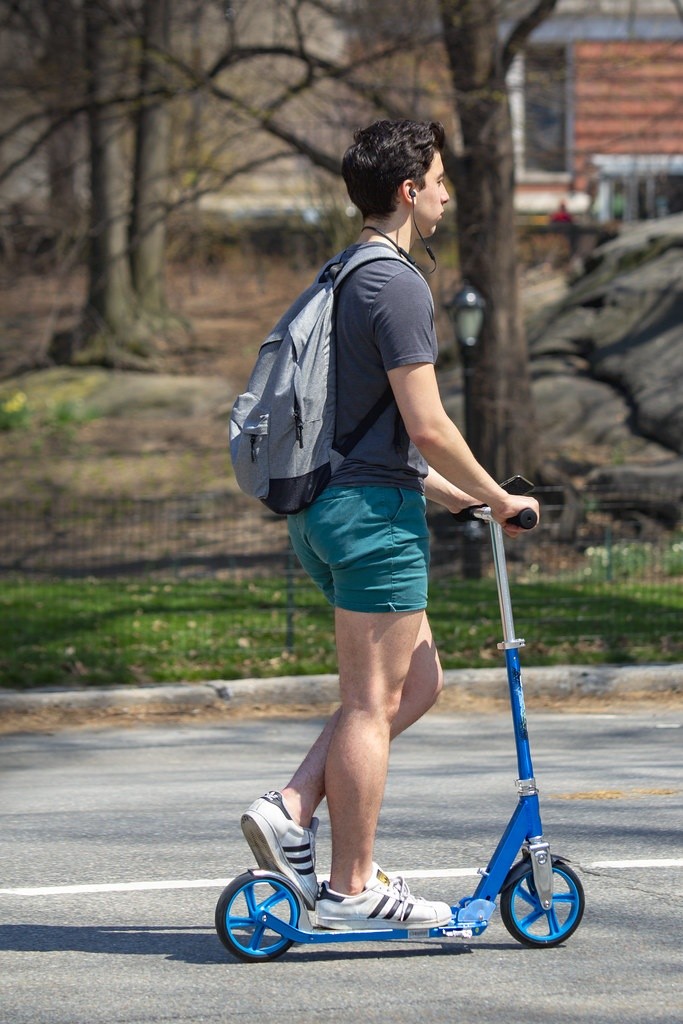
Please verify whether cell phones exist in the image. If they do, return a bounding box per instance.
[499,475,534,495]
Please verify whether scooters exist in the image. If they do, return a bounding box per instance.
[215,504,585,964]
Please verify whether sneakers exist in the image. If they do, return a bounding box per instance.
[240,790,319,910]
[315,861,452,930]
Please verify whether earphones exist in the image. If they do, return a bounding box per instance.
[409,187,415,197]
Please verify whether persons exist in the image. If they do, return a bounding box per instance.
[239,118,546,931]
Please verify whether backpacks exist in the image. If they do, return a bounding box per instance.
[228,246,435,515]
